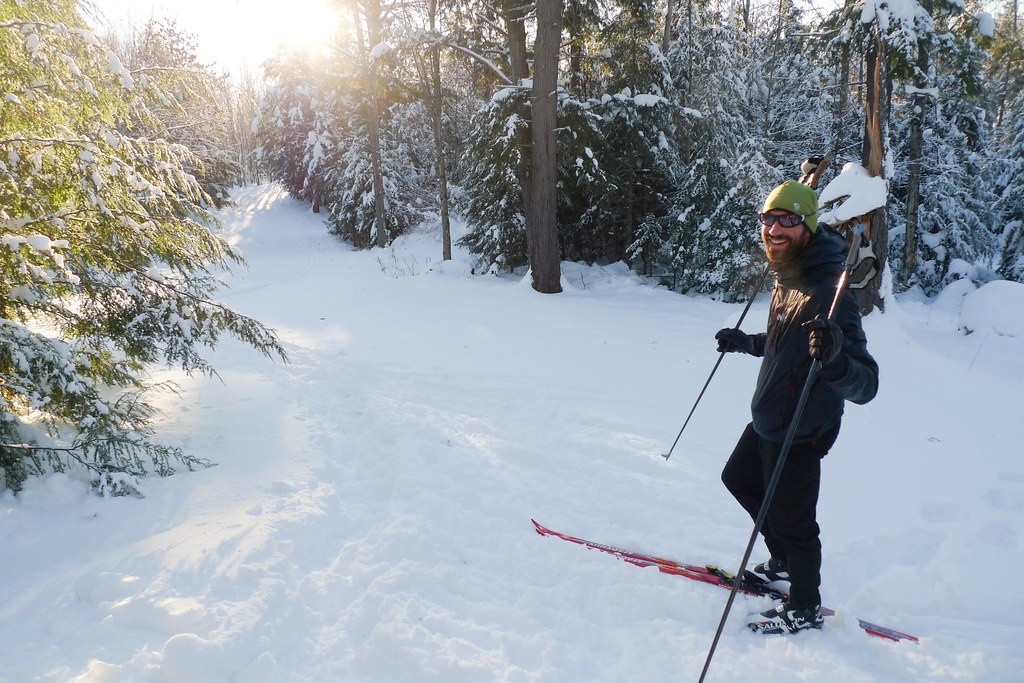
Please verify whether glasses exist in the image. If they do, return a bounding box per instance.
[758,208,805,227]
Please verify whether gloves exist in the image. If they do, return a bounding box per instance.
[715,327,747,355]
[802,315,843,365]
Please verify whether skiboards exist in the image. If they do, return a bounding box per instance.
[530,516,919,644]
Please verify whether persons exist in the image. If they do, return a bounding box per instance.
[714,181,879,635]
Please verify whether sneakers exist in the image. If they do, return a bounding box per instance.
[744,557,792,585]
[748,601,824,634]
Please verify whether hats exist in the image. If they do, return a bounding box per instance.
[763,180,818,234]
